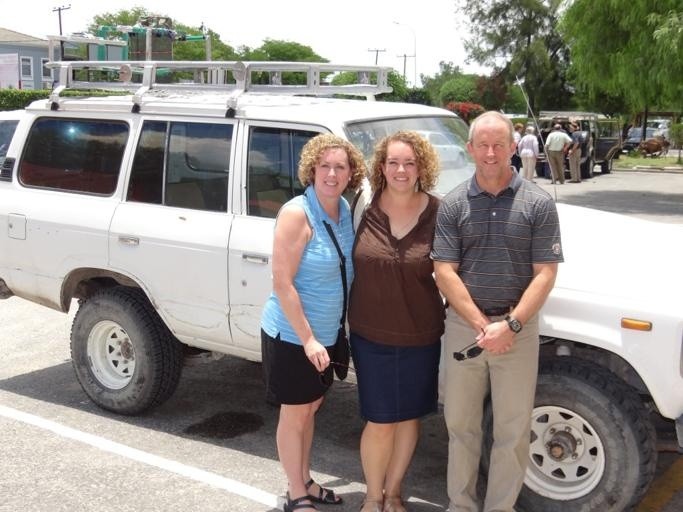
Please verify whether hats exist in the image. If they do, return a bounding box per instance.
[569,123,579,130]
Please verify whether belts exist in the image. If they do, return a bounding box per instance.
[482,306,514,317]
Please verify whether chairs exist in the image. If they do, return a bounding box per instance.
[164,173,290,218]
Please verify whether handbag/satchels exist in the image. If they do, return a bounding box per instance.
[334,320,349,380]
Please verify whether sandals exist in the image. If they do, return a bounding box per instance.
[283,478,343,512]
[358,496,406,512]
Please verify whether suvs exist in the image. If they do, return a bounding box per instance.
[0,110,35,299]
[0,53,683,512]
[532,107,621,182]
[642,118,676,152]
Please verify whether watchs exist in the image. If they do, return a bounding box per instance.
[505,315,523,335]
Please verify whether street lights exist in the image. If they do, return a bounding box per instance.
[51,4,73,61]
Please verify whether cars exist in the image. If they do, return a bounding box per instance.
[620,125,659,154]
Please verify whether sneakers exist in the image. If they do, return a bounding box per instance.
[568,180,581,183]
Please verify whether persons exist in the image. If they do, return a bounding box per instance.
[257,132,358,512]
[567,123,585,184]
[512,122,523,171]
[544,122,573,183]
[345,131,445,510]
[517,126,541,185]
[429,107,566,511]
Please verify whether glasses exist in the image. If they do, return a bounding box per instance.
[453,328,488,361]
[318,358,360,390]
